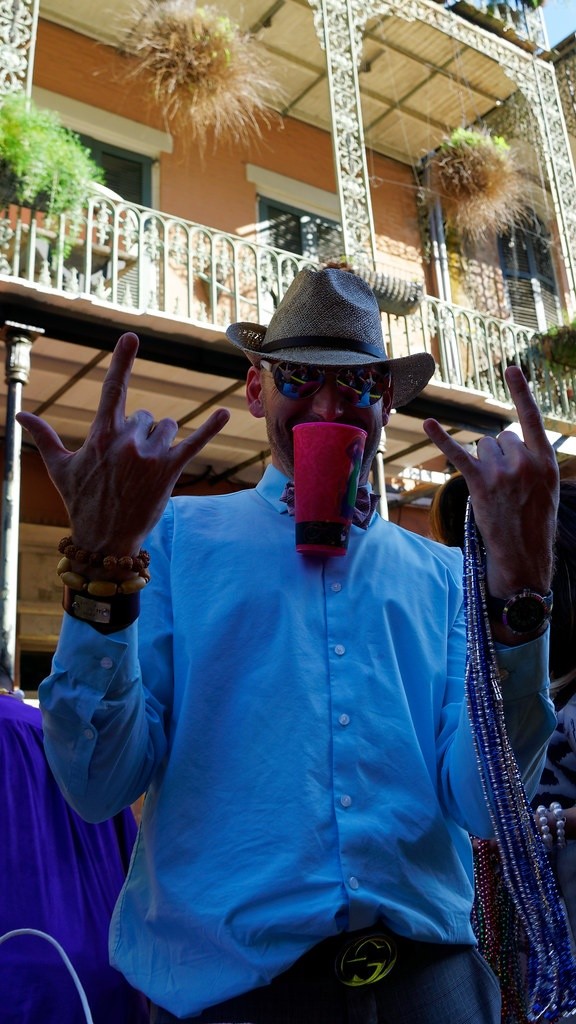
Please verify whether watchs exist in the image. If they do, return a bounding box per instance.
[485,588,554,639]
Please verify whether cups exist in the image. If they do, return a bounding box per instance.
[292,422,367,557]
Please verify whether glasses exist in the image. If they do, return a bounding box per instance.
[260,359,392,409]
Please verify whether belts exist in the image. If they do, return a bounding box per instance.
[275,933,465,988]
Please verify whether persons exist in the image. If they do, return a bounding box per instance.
[16,265,560,1024]
[428,472,576,1024]
[0,628,138,1024]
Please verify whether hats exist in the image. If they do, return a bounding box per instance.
[225,264,435,410]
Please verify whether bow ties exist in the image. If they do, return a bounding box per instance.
[279,481,381,531]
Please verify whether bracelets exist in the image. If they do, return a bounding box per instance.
[59,585,140,625]
[548,802,568,849]
[53,536,150,573]
[537,805,554,852]
[56,558,151,595]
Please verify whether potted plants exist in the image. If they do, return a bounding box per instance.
[1,94,139,272]
